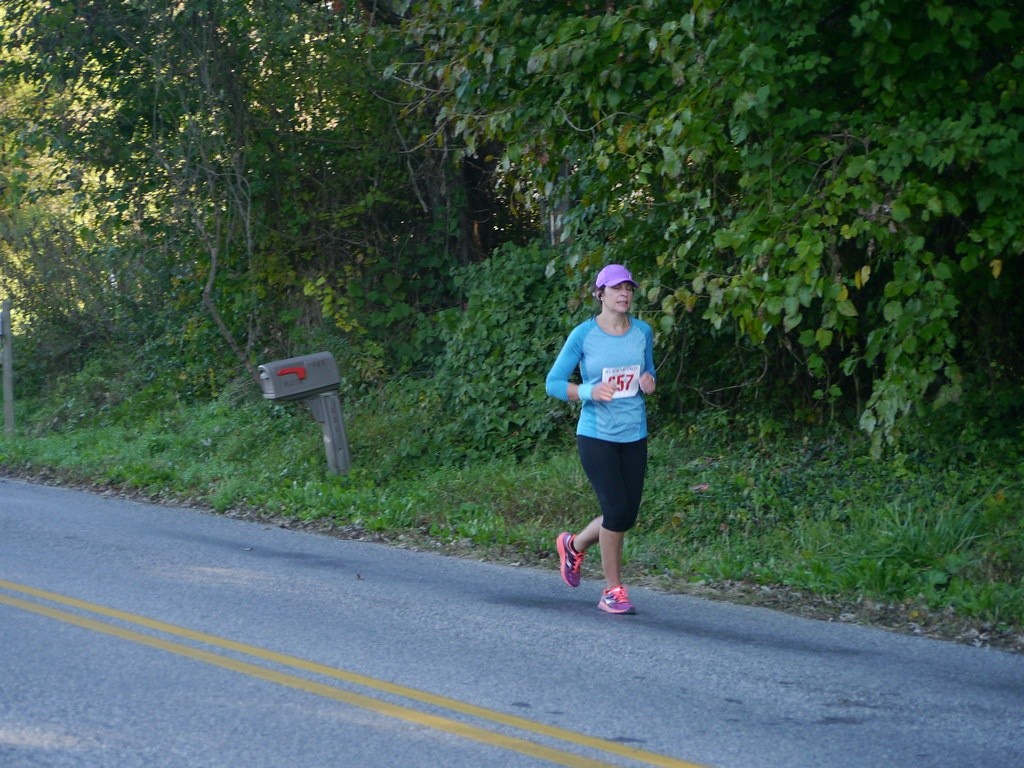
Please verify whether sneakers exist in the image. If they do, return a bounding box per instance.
[556,532,587,587]
[598,585,636,613]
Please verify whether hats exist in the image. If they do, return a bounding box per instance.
[595,264,639,288]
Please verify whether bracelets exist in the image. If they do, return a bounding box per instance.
[651,377,655,382]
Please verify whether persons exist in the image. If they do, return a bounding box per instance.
[545,264,656,614]
